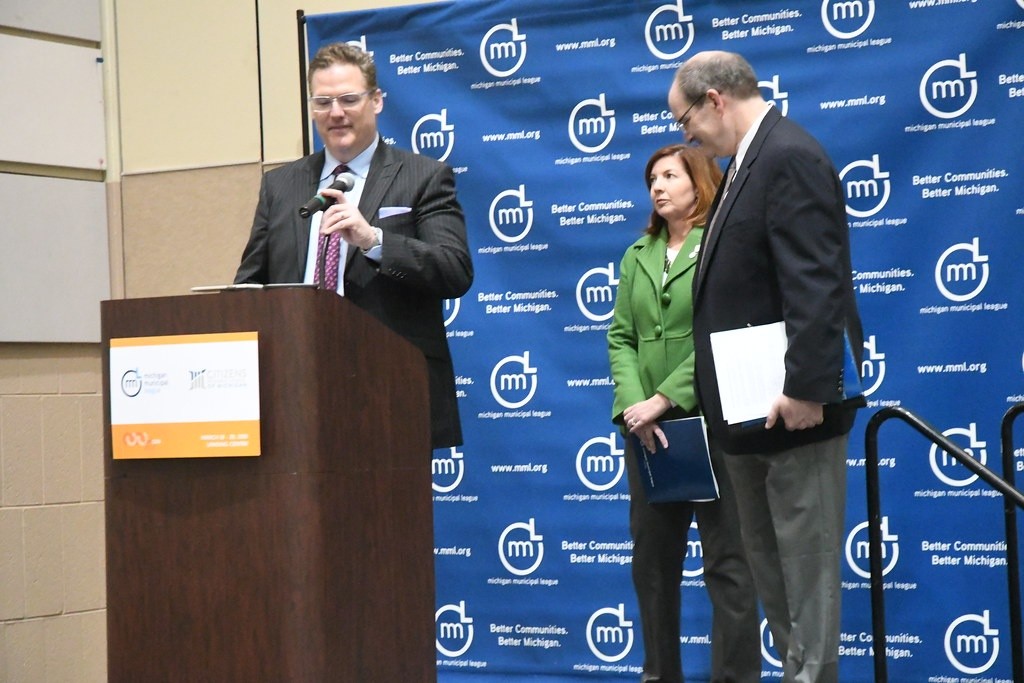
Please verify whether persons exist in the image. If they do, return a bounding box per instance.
[666,47,868,683]
[228,44,475,464]
[606,145,762,683]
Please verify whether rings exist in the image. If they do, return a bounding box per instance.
[631,417,637,426]
[340,211,345,220]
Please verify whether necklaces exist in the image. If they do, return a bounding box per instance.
[664,242,671,274]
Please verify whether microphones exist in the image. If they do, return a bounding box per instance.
[299,172,357,220]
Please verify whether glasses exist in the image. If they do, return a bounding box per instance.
[309,90,370,112]
[676,94,706,133]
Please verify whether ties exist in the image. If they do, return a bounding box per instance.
[697,159,736,280]
[314,165,349,292]
